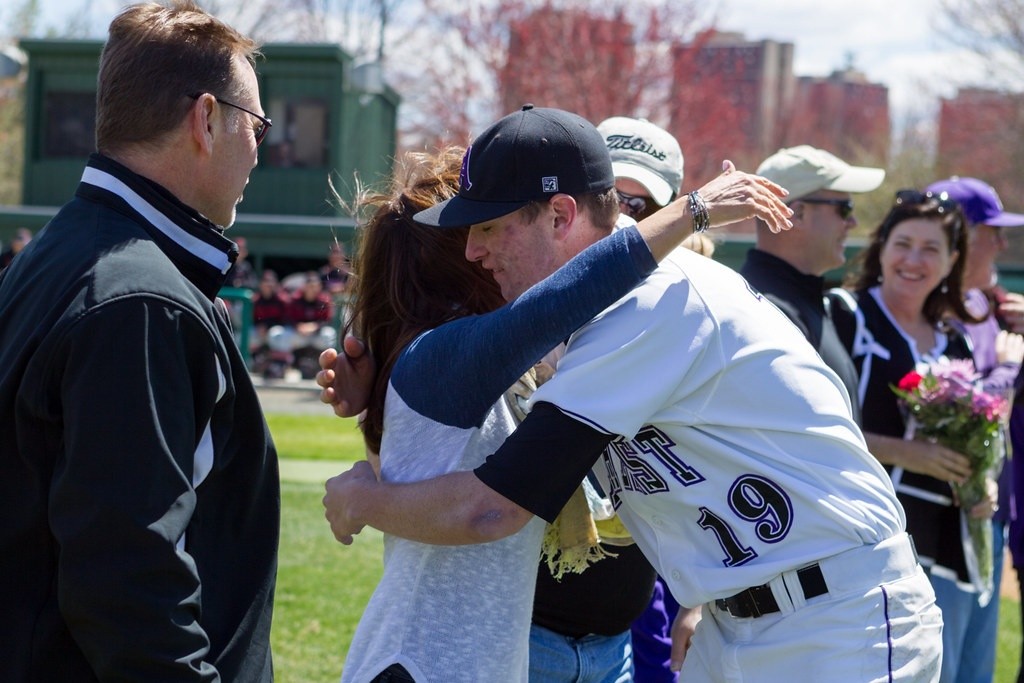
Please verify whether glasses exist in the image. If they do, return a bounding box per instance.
[896,190,956,215]
[187,90,272,147]
[617,190,663,222]
[801,199,854,219]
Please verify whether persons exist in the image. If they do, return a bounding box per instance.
[0,0,281,683]
[252,103,1024,683]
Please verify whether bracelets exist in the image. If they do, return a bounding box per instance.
[683,190,710,233]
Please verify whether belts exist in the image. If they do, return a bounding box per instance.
[533,613,612,642]
[715,535,920,618]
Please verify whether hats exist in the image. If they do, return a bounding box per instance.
[597,117,684,206]
[756,145,886,204]
[925,175,1024,227]
[413,103,614,226]
